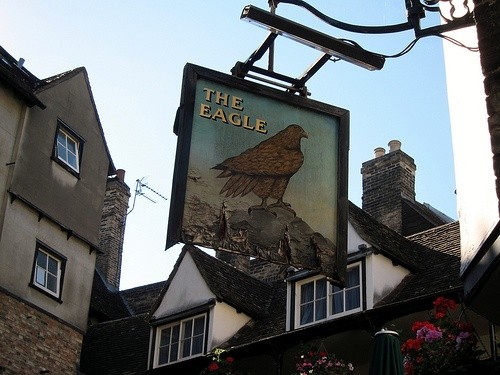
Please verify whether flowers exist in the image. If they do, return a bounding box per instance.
[404,294,478,374]
[297,350,356,375]
